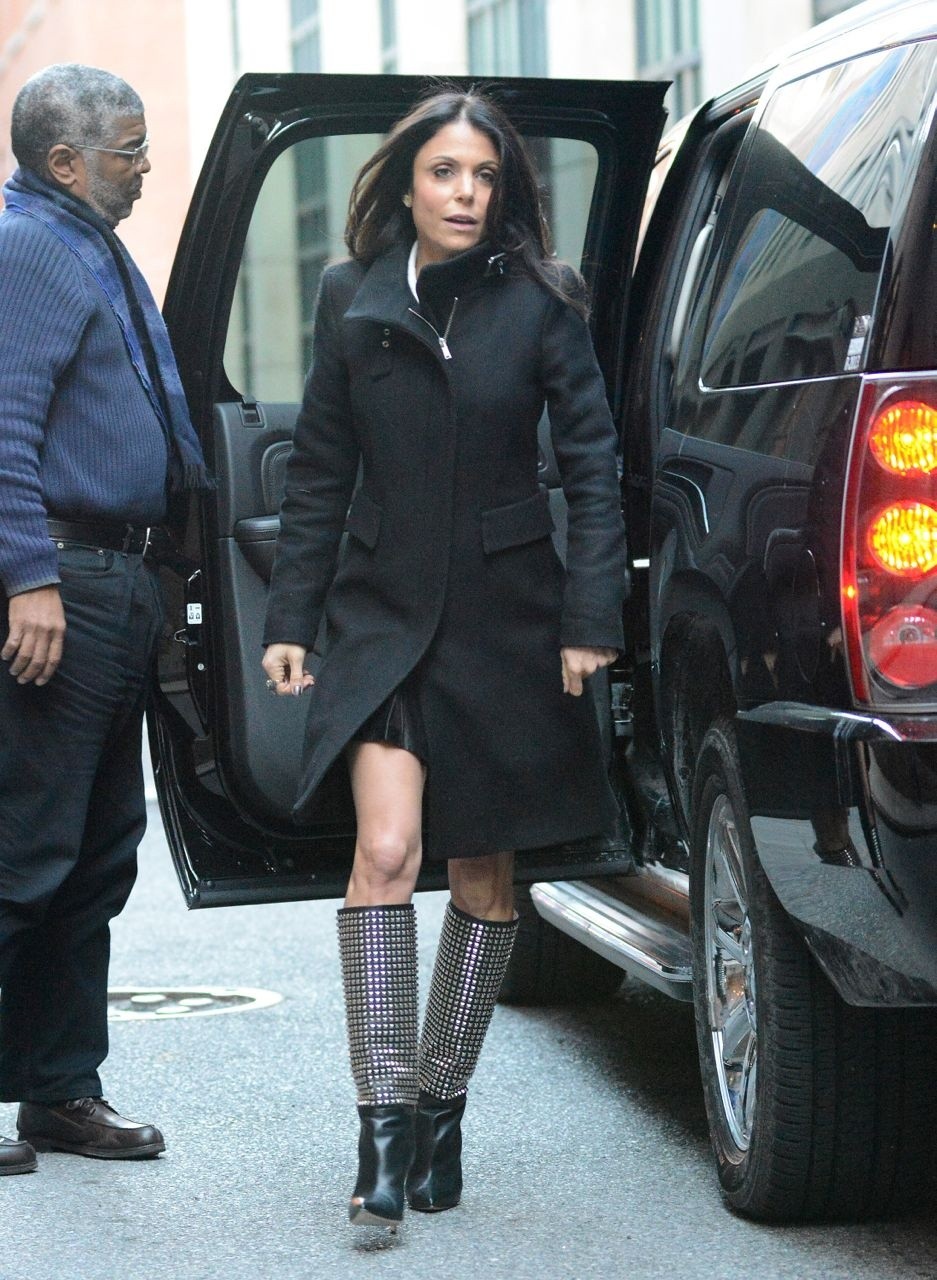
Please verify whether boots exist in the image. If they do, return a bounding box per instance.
[336,903,418,1230]
[407,901,519,1213]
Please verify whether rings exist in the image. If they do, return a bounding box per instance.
[266,679,278,697]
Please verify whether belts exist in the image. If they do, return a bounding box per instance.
[46,520,174,562]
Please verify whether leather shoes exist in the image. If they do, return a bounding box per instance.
[0,1136,38,1175]
[15,1098,166,1159]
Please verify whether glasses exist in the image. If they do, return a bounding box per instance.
[78,144,148,164]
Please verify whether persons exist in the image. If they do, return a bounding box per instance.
[1,64,207,1176]
[262,91,630,1233]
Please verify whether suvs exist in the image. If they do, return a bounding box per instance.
[131,0,937,1231]
